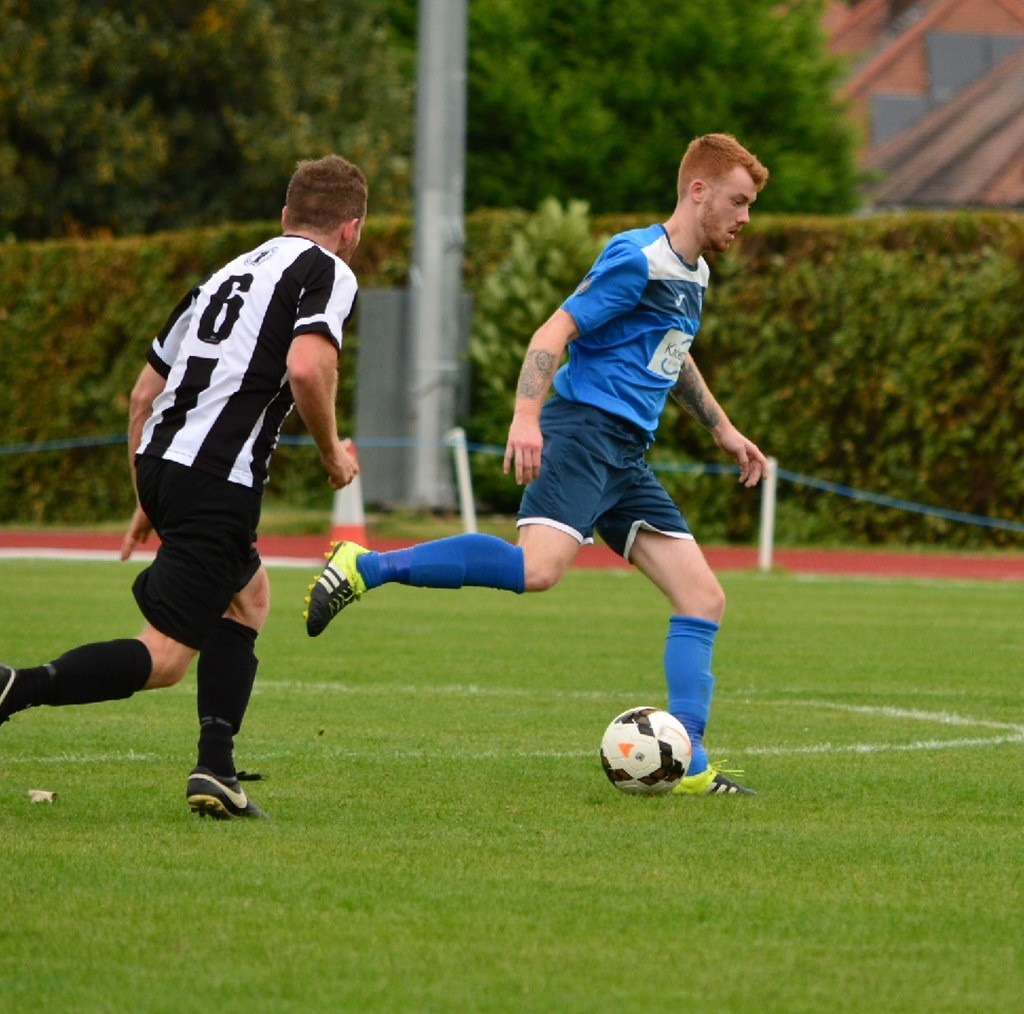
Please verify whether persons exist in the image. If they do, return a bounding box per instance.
[0,152,369,826]
[302,135,773,802]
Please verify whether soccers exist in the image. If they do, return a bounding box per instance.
[600,705,691,793]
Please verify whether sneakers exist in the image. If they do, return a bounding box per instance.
[672,766,760,797]
[0,665,19,727]
[302,539,371,637]
[184,767,270,821]
